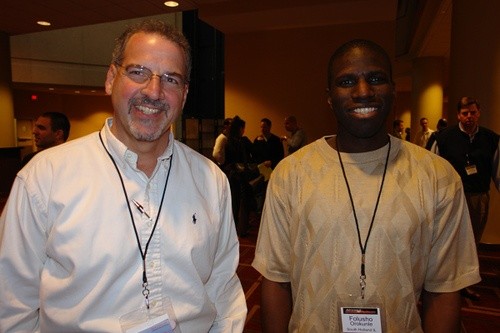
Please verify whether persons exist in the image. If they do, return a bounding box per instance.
[252,39,481,333]
[426,97,500,244]
[393,118,434,142]
[0,18,246,333]
[32,112,71,149]
[212,116,306,239]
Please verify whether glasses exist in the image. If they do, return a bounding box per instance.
[115,60,190,93]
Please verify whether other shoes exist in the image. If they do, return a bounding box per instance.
[461,287,480,300]
